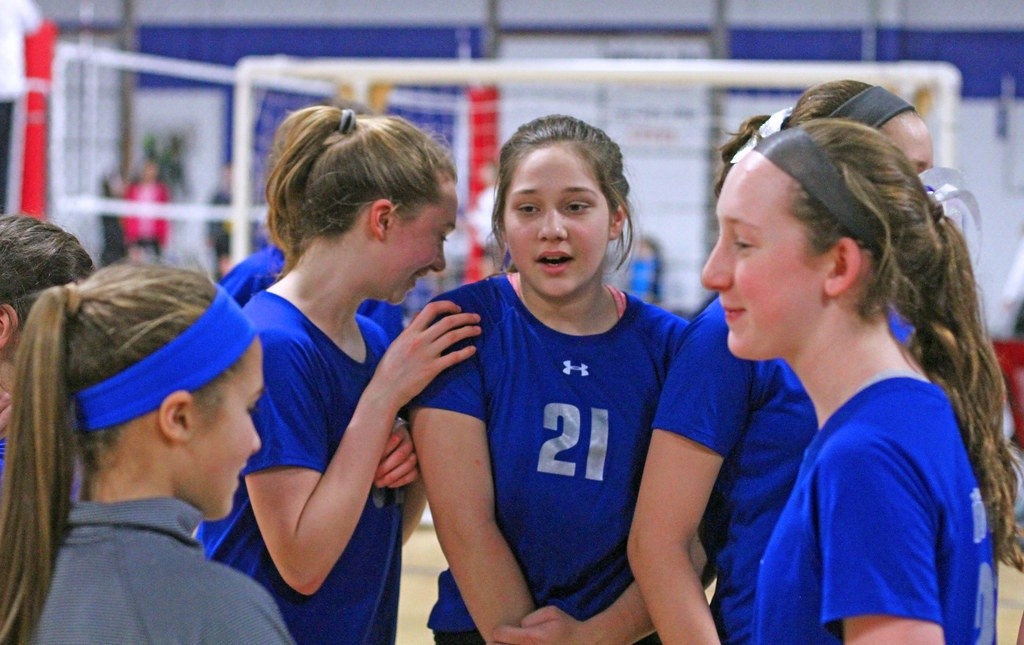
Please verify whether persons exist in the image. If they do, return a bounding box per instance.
[699,120,1024,645]
[0,78,933,645]
